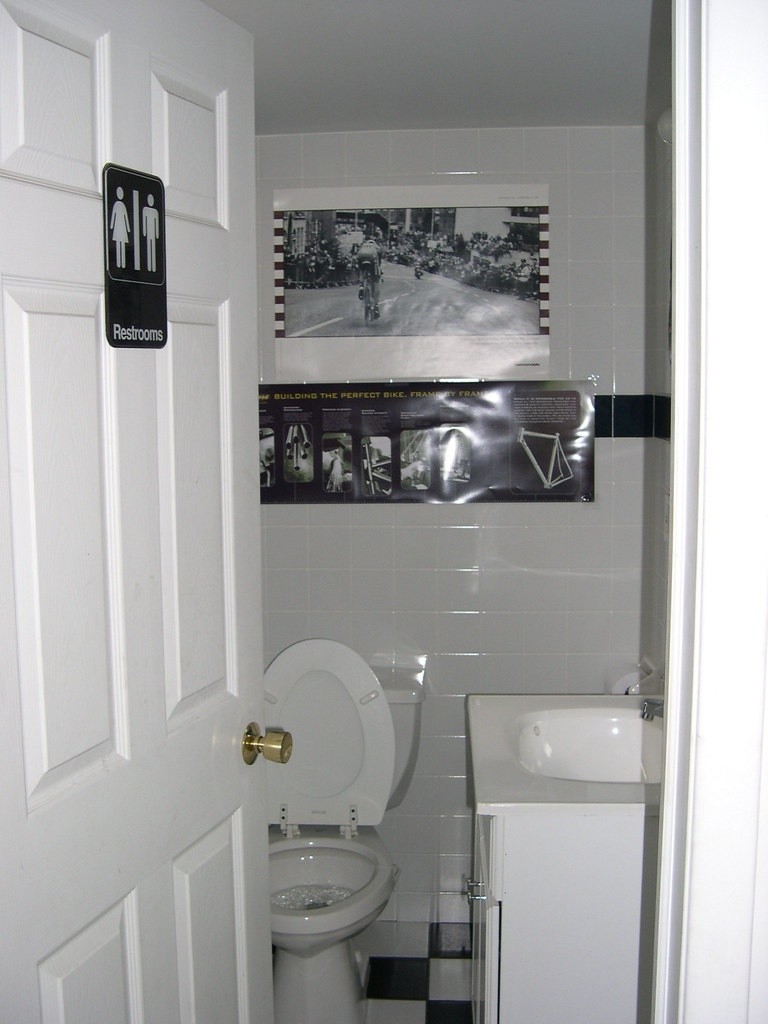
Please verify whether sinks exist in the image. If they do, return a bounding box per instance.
[504,708,664,785]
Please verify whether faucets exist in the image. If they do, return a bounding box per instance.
[640,698,664,722]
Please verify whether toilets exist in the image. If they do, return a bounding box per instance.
[262,637,425,1024]
